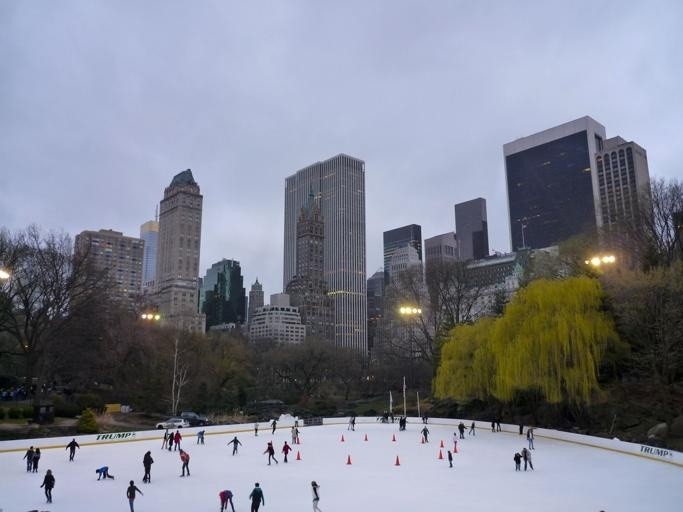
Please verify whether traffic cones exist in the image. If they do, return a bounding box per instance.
[363,433,369,441]
[340,434,344,442]
[294,450,300,460]
[296,437,299,444]
[438,440,457,459]
[394,455,400,465]
[391,434,397,443]
[346,455,352,464]
[421,436,425,443]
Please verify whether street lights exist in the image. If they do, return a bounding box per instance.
[139,313,160,382]
[399,307,421,386]
[583,251,615,274]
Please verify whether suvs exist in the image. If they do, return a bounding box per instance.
[178,411,208,426]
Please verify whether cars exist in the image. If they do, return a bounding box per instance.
[155,417,189,430]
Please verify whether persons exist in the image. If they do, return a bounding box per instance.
[1,383,46,398]
[228,435,241,455]
[281,441,292,462]
[41,470,55,503]
[249,483,265,512]
[348,410,535,471]
[96,466,114,480]
[127,481,142,511]
[254,421,258,435]
[143,428,205,483]
[312,480,321,512]
[271,419,276,434]
[291,416,299,443]
[264,440,278,465]
[31,448,40,472]
[66,438,79,460]
[218,490,235,512]
[23,446,34,472]
